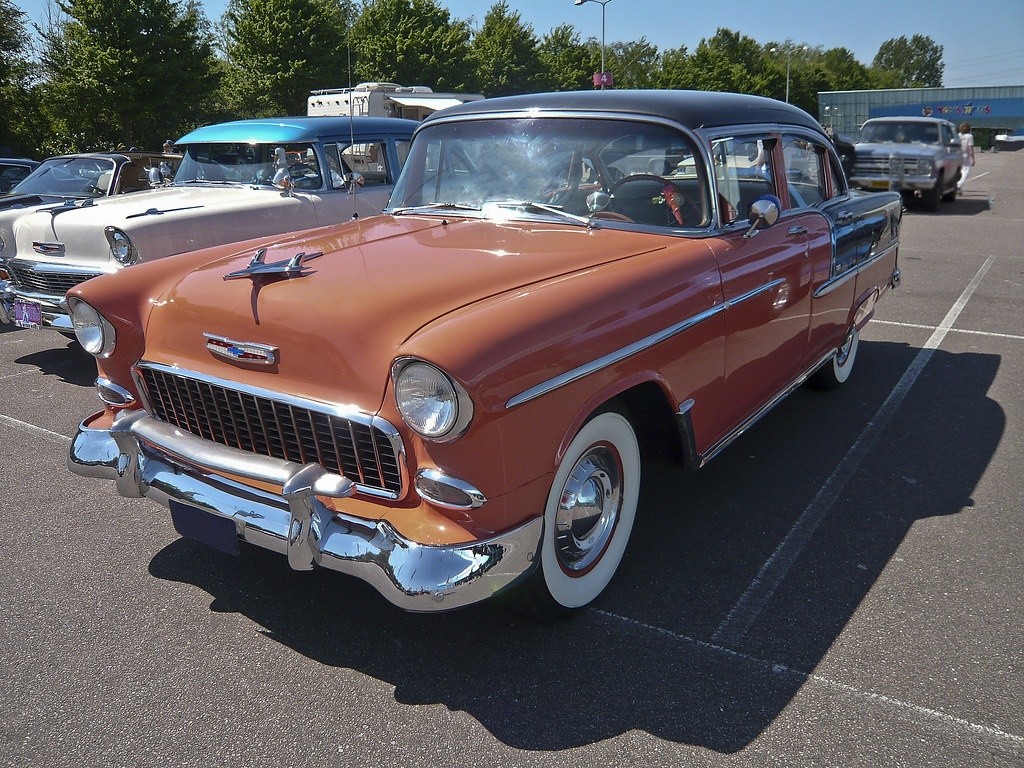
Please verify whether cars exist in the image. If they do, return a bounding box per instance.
[0,116,488,358]
[604,129,811,185]
[66,87,907,622]
[1,151,243,211]
[849,115,962,211]
[0,157,96,207]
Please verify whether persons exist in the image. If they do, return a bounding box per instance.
[956,121,975,195]
[117,143,126,152]
[273,145,287,169]
[160,140,174,168]
[754,140,773,180]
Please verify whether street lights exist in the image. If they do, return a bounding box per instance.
[574,0,612,90]
[769,45,809,104]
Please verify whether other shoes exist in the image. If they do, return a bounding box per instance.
[957,186,965,196]
